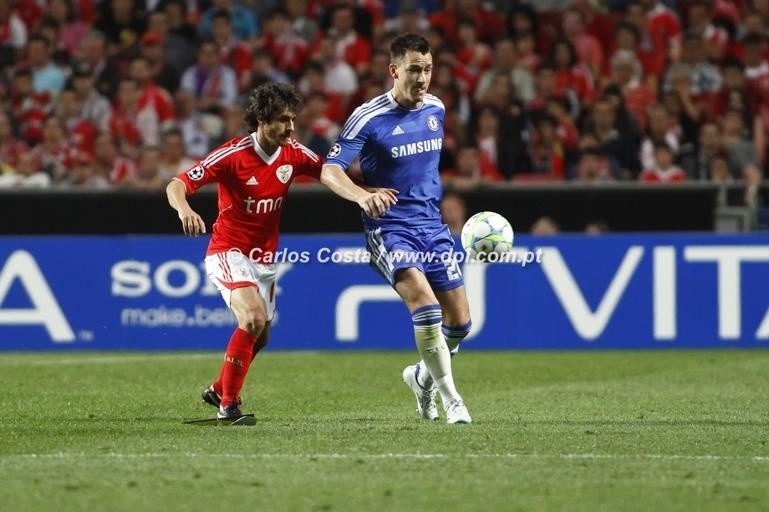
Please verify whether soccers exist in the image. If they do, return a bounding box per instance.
[462,211,513,263]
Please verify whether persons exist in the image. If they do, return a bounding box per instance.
[320,32,471,424]
[166,82,400,425]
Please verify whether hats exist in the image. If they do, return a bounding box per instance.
[139,29,163,48]
[71,60,93,79]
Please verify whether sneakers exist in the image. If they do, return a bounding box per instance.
[446,399,472,424]
[201,385,219,409]
[402,363,439,421]
[217,404,243,423]
